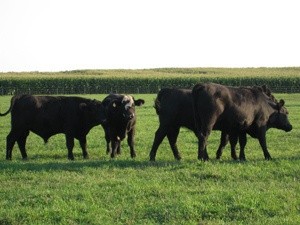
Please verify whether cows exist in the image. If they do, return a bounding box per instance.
[102,92,145,159]
[190,82,292,160]
[0,92,111,161]
[149,83,279,162]
